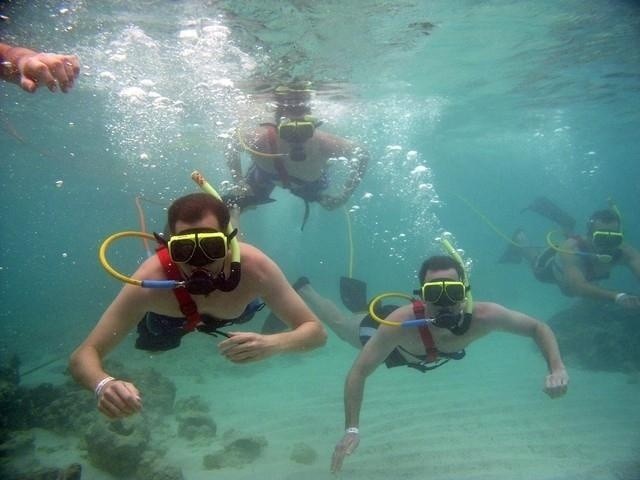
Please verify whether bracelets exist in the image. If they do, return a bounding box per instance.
[615,292,626,305]
[95,376,115,398]
[345,428,359,434]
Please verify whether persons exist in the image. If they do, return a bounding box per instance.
[507,206,640,311]
[0,42,81,94]
[67,193,328,419]
[292,255,569,472]
[225,90,369,212]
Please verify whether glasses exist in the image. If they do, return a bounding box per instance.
[422,282,466,304]
[591,231,625,252]
[278,122,315,141]
[168,232,228,264]
